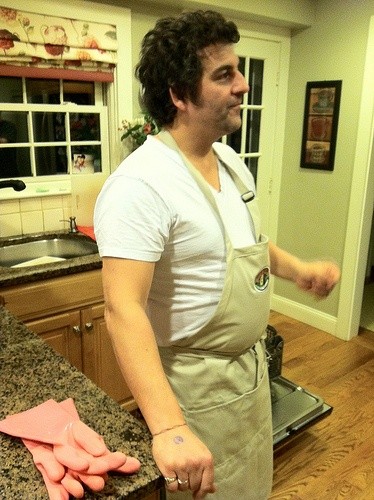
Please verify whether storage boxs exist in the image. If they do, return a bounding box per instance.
[265,324,283,379]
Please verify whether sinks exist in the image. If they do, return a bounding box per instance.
[0,229,103,281]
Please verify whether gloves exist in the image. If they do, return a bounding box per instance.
[0,398,141,500]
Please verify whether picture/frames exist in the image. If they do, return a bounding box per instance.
[300,79,344,172]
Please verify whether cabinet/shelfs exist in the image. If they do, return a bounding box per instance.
[0,265,169,499]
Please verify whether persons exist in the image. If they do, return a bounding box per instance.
[73,154,88,174]
[92,9,340,499]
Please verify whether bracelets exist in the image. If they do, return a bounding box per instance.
[152,423,188,438]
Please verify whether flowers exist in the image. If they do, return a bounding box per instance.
[116,115,156,146]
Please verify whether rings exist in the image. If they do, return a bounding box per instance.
[164,477,177,484]
[177,478,189,485]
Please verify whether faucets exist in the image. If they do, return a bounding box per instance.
[59,215,79,233]
